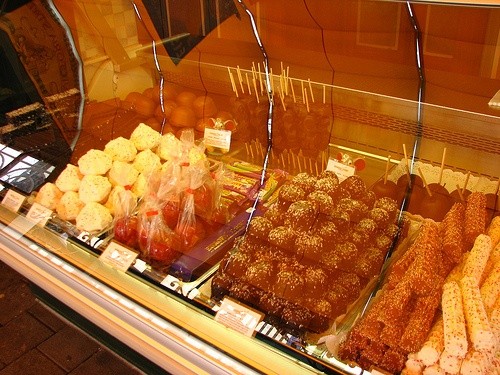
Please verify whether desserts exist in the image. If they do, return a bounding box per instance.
[33,123,500,375]
